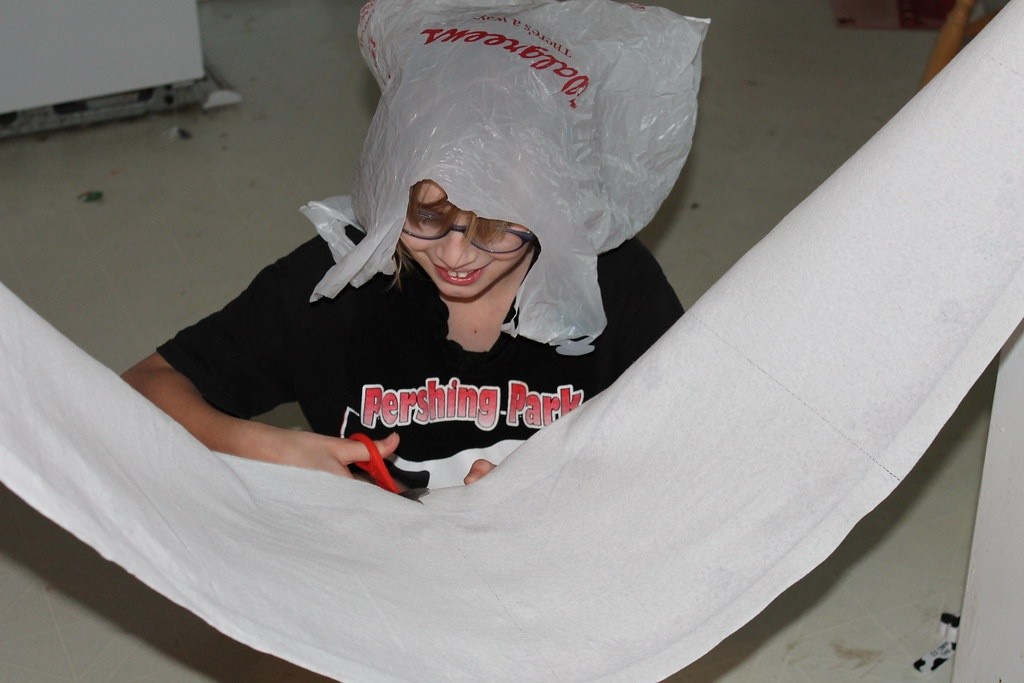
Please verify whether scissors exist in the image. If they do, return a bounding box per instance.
[349,433,430,506]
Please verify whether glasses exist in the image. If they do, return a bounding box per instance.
[397,206,536,254]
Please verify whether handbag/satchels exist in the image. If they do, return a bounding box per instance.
[300,0,711,355]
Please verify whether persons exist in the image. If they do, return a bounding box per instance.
[114,180,684,492]
